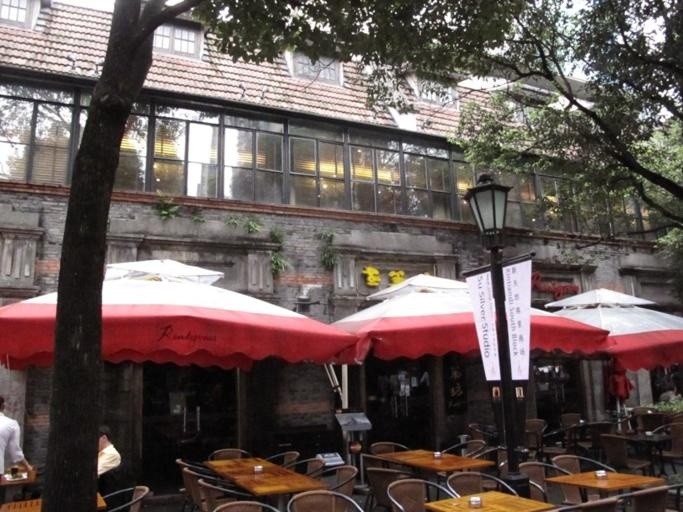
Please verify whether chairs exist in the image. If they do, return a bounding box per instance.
[177,439,361,511]
[98,480,156,511]
[363,418,682,511]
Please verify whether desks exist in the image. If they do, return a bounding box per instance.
[422,492,558,512]
[0,464,38,500]
[364,444,496,489]
[547,466,666,503]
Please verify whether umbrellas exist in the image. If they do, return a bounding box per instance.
[544,287,683,371]
[1,258,359,467]
[330,271,610,360]
[607,362,634,435]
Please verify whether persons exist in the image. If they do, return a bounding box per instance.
[0,394,32,505]
[659,385,681,402]
[97,425,121,478]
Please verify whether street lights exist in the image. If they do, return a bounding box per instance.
[462,173,538,498]
